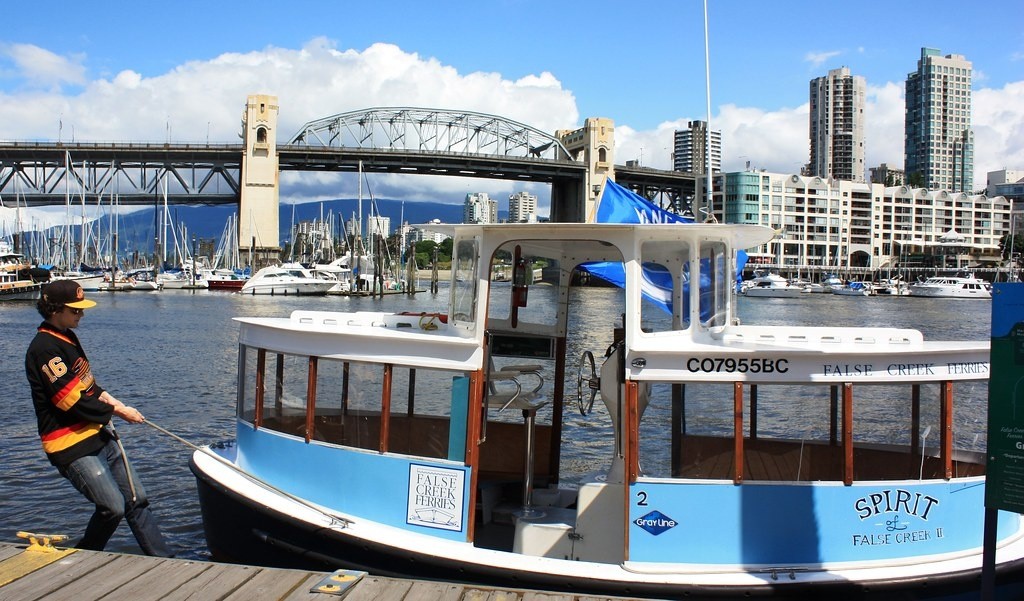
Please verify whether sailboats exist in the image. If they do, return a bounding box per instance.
[320,157,428,295]
[0,150,249,302]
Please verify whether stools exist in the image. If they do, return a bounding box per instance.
[462,332,554,520]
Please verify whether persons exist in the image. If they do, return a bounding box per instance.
[24,279,176,559]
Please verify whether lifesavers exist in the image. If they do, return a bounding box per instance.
[120,278,126,283]
[22,269,29,275]
[105,274,110,280]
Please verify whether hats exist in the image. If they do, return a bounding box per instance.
[41,280,97,308]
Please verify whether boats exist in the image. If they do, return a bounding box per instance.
[240,260,343,300]
[905,266,992,301]
[806,246,908,297]
[188,1,1024,599]
[741,266,803,298]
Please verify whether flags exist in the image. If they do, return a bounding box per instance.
[578,180,749,325]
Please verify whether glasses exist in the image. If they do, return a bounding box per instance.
[64,306,84,314]
